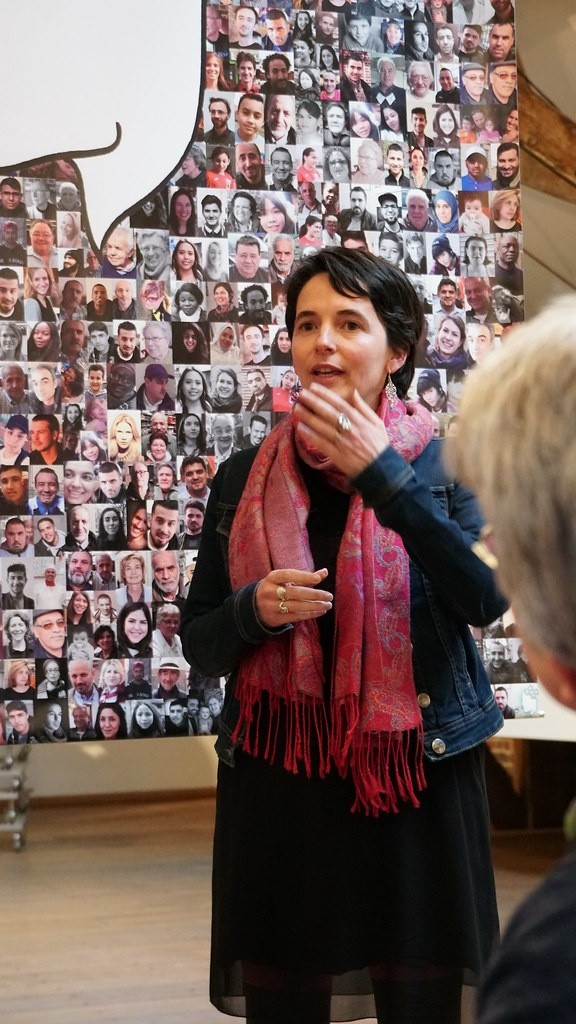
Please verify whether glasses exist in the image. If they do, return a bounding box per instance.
[492,70,518,81]
[30,233,54,239]
[35,618,65,629]
[1,191,21,197]
[463,74,486,81]
[109,371,134,384]
[142,336,165,342]
[45,666,59,674]
[140,293,164,302]
[206,16,221,22]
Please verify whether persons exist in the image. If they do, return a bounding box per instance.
[114,280,138,319]
[241,327,271,366]
[208,369,242,412]
[438,294,575,1024]
[137,364,175,410]
[176,414,205,457]
[94,508,126,552]
[26,415,61,465]
[29,366,61,413]
[109,413,139,462]
[180,244,512,1024]
[243,370,272,411]
[209,283,239,322]
[0,0,538,743]
[35,518,67,555]
[94,626,118,659]
[33,608,67,658]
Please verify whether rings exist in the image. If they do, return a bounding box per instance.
[339,413,353,431]
[279,602,288,614]
[277,586,286,599]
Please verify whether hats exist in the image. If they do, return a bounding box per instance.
[175,283,203,306]
[490,60,517,71]
[66,250,84,264]
[3,221,17,232]
[5,415,29,435]
[33,592,64,617]
[432,234,449,248]
[417,369,441,386]
[378,192,398,205]
[145,364,174,379]
[153,657,188,670]
[184,550,198,567]
[463,145,488,162]
[462,63,488,75]
